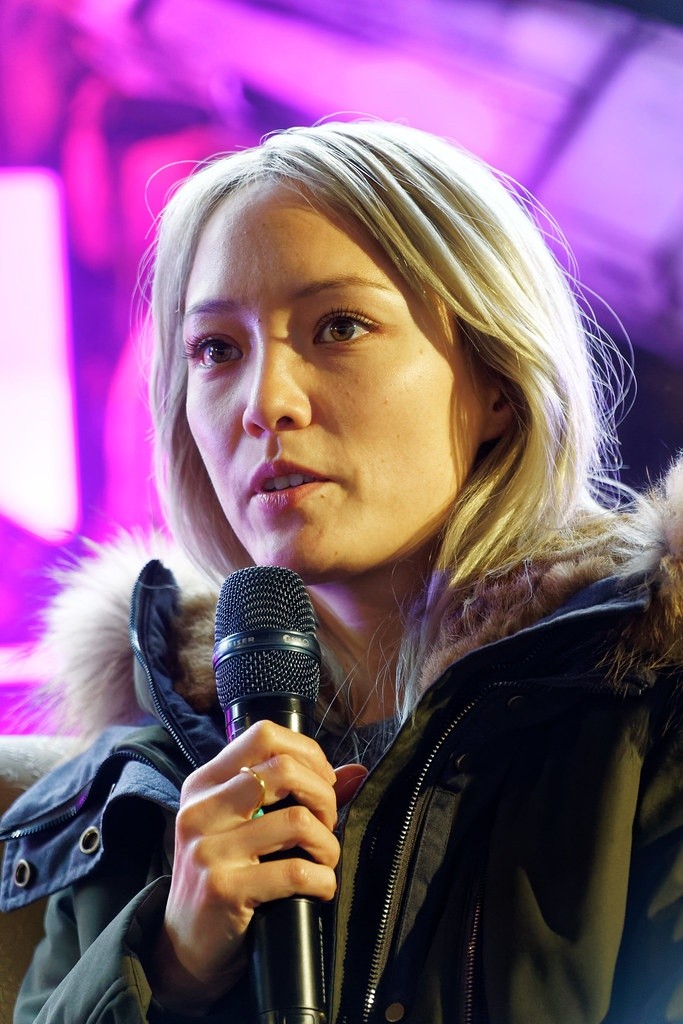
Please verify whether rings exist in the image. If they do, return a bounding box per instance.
[237,765,267,816]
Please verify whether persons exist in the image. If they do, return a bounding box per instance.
[0,109,683,1024]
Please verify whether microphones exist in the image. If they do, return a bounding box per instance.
[212,563,327,1023]
